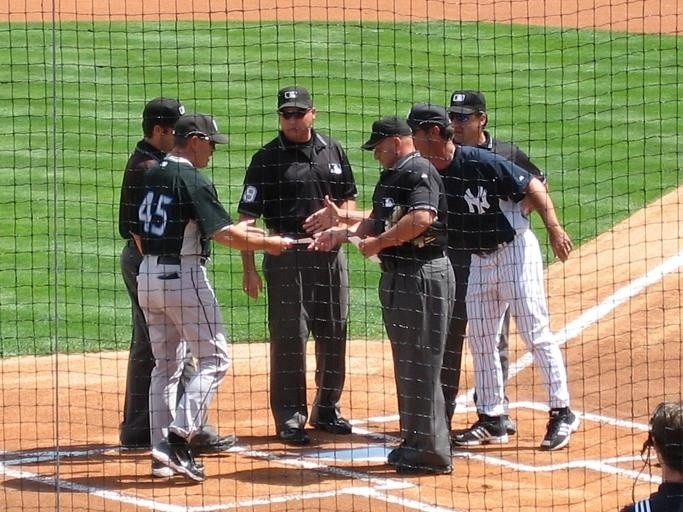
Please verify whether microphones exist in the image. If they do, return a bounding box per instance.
[640,441,661,467]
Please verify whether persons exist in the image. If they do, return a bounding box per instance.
[138,113,293,481]
[118,96,239,452]
[302,101,580,451]
[618,400,683,511]
[236,86,358,445]
[441,90,549,443]
[308,115,456,474]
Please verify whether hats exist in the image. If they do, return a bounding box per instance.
[276,86,312,110]
[172,113,228,144]
[446,90,485,114]
[361,118,412,150]
[144,97,185,119]
[407,104,449,134]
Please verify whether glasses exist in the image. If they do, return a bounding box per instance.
[277,108,311,120]
[448,112,473,123]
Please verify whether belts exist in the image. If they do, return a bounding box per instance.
[471,236,514,255]
[157,256,205,265]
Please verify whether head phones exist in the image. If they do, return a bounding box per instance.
[639,402,683,447]
